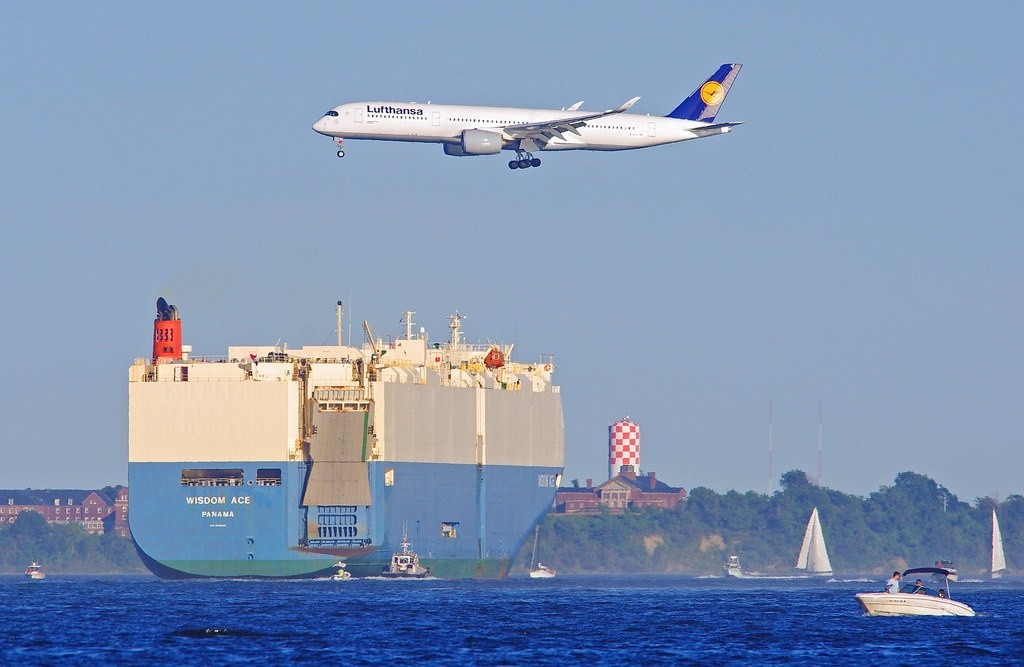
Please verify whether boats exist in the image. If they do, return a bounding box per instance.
[25,562,43,576]
[529,563,557,581]
[381,523,428,578]
[723,554,744,577]
[31,573,47,580]
[852,560,977,617]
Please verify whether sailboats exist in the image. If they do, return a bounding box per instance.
[792,506,834,575]
[990,507,1008,579]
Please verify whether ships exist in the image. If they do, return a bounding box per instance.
[124,296,567,581]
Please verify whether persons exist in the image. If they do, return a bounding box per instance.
[912,579,925,595]
[886,572,900,594]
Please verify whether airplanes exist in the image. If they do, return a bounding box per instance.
[312,62,744,171]
